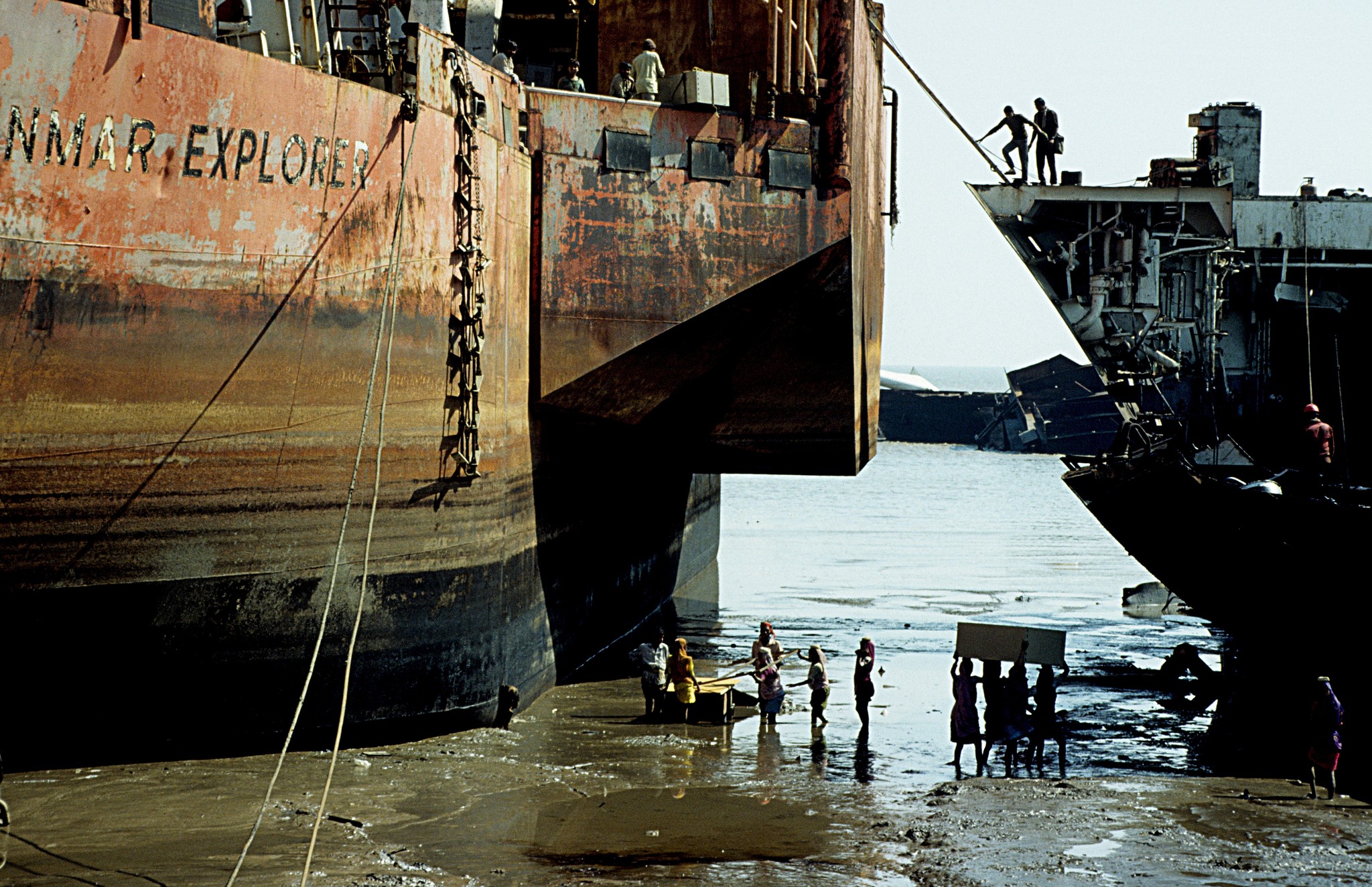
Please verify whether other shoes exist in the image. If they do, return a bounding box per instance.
[1004,168,1016,175]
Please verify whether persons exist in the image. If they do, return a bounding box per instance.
[981,660,1009,770]
[948,650,983,779]
[1028,98,1059,186]
[490,40,519,84]
[1296,403,1334,464]
[627,626,670,720]
[630,38,665,101]
[974,106,1049,185]
[1004,640,1037,784]
[747,646,786,726]
[1306,677,1344,799]
[786,645,830,728]
[750,621,785,723]
[1025,658,1071,780]
[662,637,701,724]
[610,61,636,99]
[557,59,586,93]
[853,639,875,744]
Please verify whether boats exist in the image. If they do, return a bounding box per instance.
[0,0,899,778]
[866,16,1372,690]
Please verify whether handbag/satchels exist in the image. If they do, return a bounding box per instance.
[1052,132,1064,155]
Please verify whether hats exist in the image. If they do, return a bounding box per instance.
[643,38,656,51]
